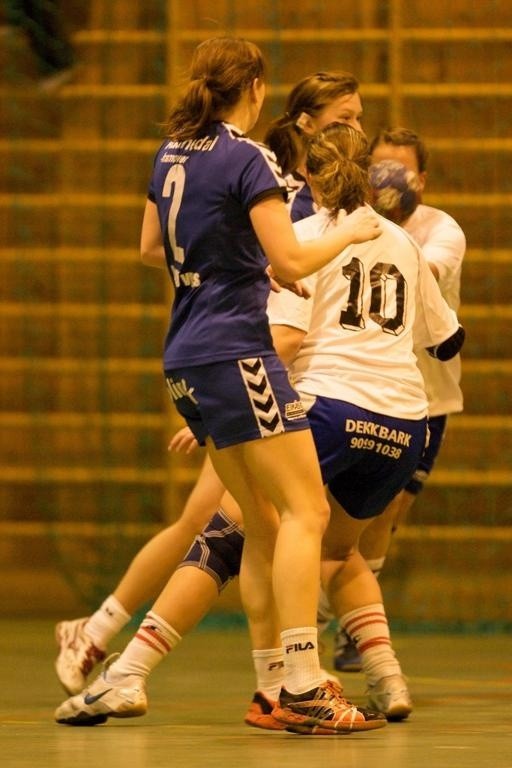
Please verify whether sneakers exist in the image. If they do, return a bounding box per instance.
[244,625,412,735]
[55,670,147,726]
[56,618,106,694]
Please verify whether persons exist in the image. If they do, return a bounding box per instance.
[52,67,371,698]
[136,30,391,739]
[331,121,467,674]
[51,116,468,728]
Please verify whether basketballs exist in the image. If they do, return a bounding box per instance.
[366,160,420,225]
